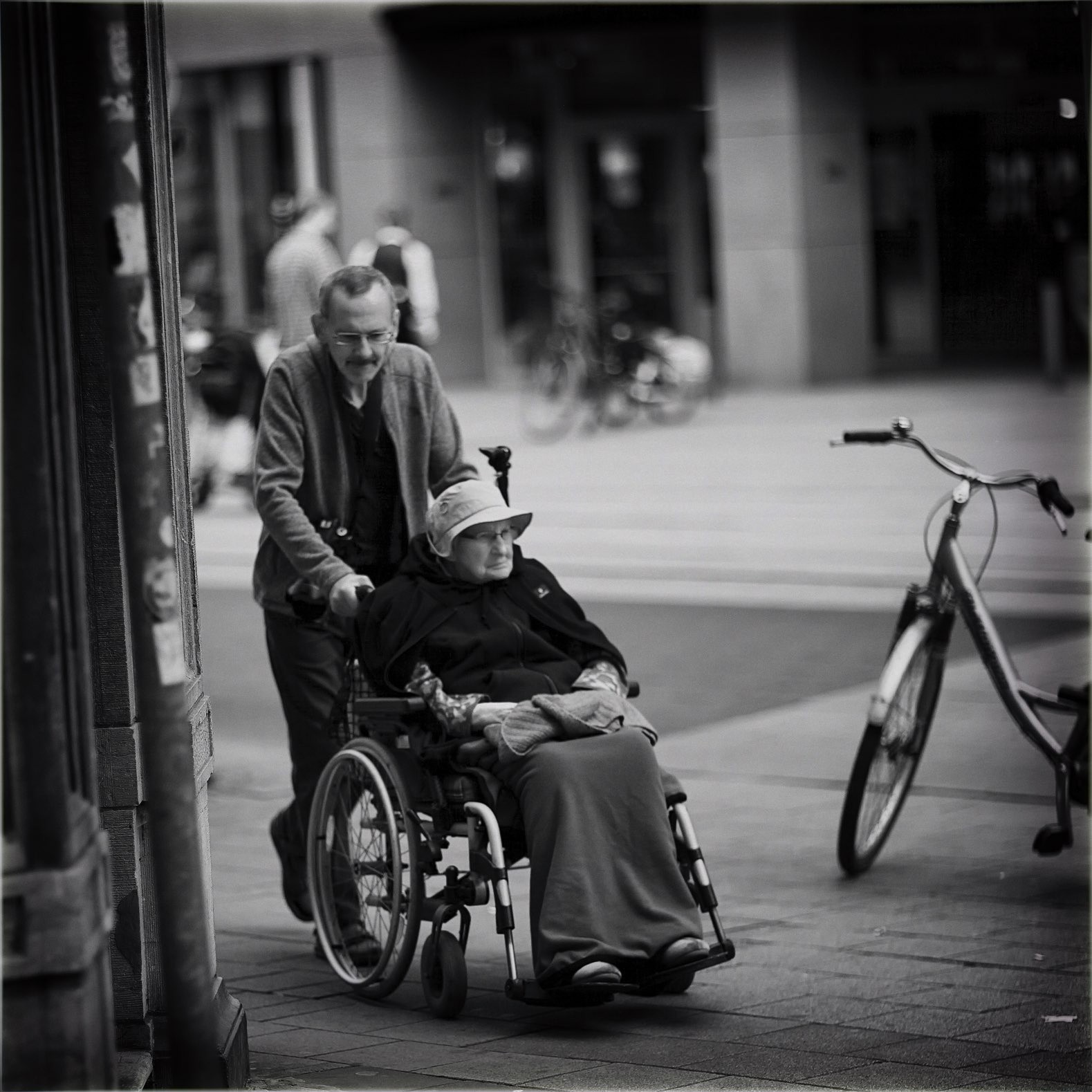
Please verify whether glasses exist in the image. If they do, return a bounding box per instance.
[320,312,397,345]
[462,527,515,543]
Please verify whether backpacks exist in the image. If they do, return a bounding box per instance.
[373,245,411,321]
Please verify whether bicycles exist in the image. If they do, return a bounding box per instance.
[829,414,1092,878]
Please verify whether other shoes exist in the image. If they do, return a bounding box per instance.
[574,961,622,982]
[312,919,382,965]
[271,815,314,922]
[664,937,711,959]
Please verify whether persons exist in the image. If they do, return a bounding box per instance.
[183,194,441,510]
[352,477,712,988]
[252,263,482,967]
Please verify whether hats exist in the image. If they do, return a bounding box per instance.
[425,480,534,557]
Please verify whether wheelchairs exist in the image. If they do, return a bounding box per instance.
[304,584,735,1021]
